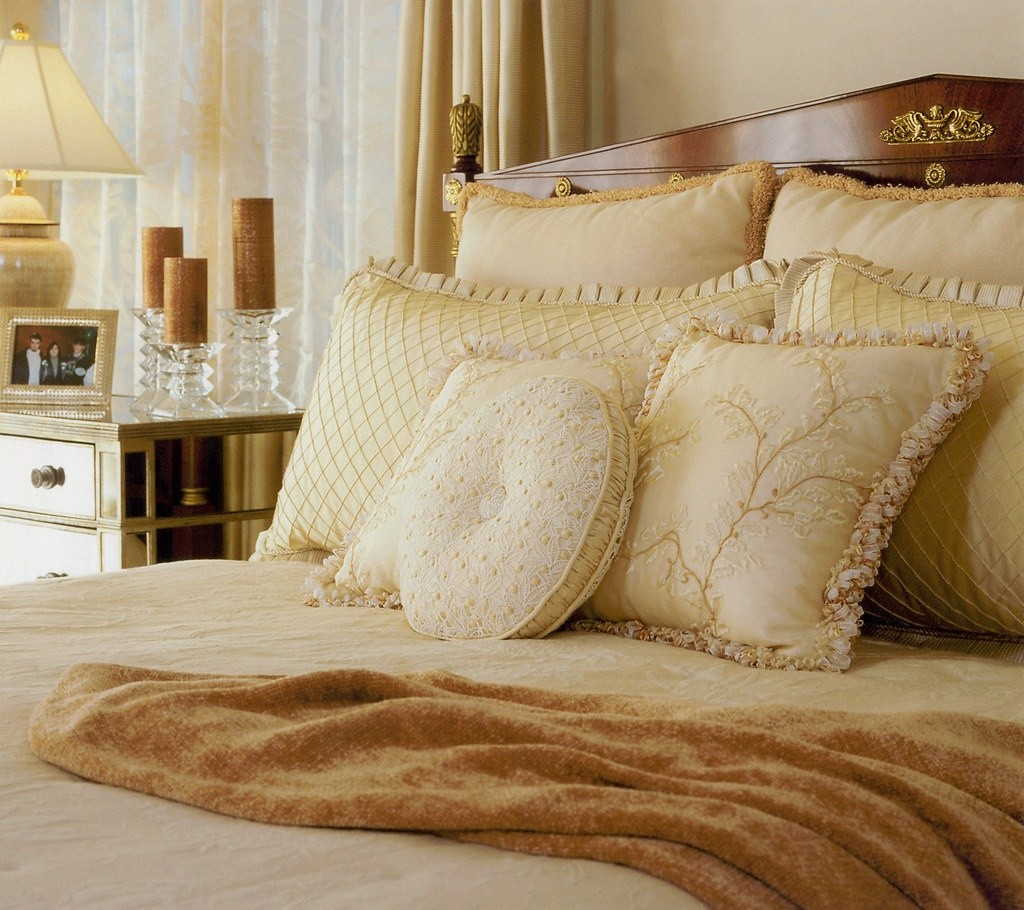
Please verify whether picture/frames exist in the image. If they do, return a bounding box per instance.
[0,307,119,407]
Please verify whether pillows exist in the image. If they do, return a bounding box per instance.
[447,158,779,287]
[257,255,790,564]
[572,309,993,671]
[763,167,1024,286]
[776,248,1024,643]
[299,334,651,616]
[396,376,640,638]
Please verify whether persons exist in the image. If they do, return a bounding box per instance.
[41,341,65,385]
[13,334,44,385]
[60,338,96,386]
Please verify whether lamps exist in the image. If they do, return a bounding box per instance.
[163,258,209,343]
[231,196,279,310]
[141,226,184,308]
[0,21,146,387]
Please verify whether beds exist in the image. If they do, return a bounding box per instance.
[0,71,1024,910]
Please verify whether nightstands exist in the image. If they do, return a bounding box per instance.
[0,393,306,585]
